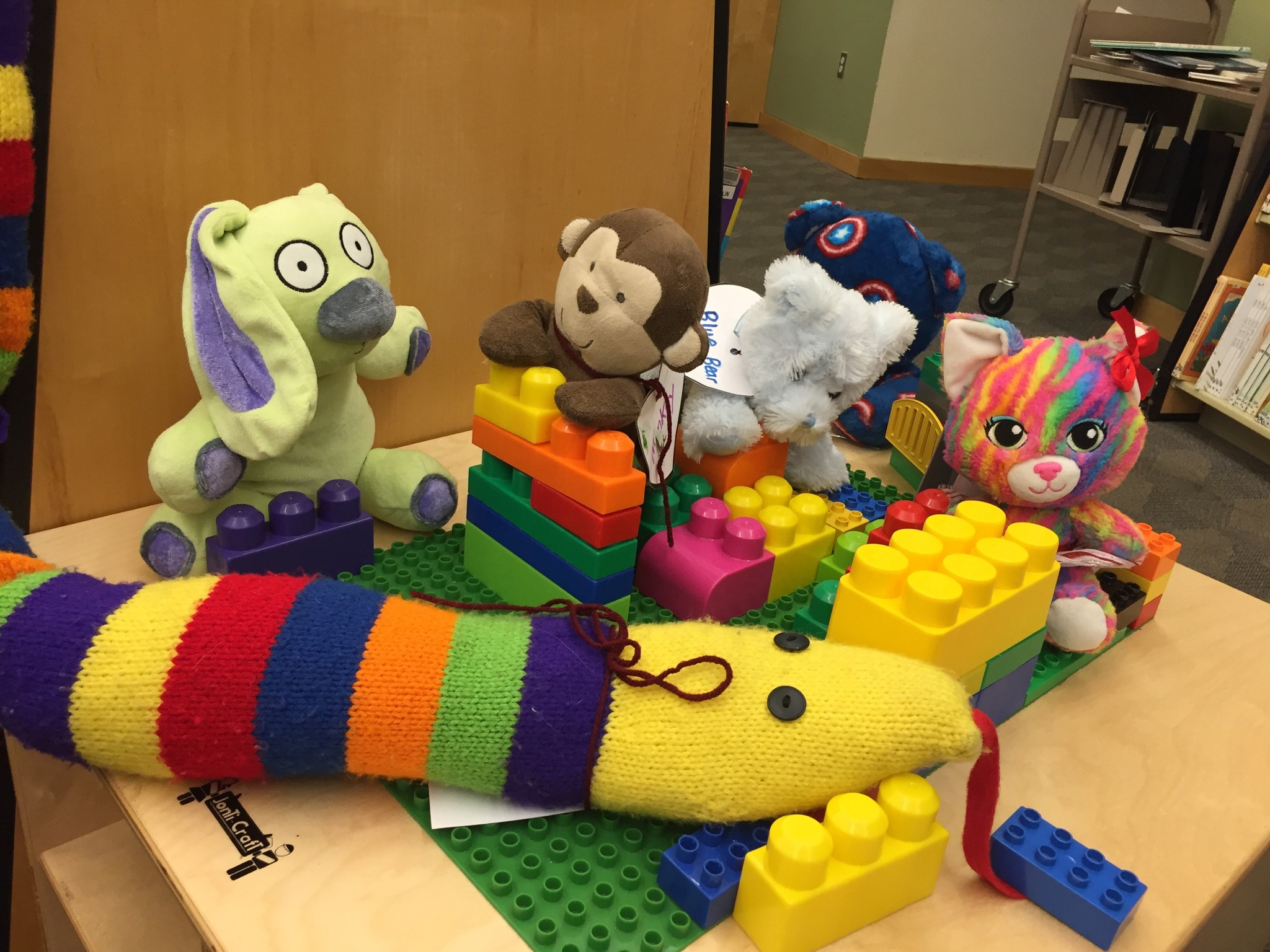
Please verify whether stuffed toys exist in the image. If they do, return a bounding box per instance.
[941,307,1160,653]
[478,206,709,466]
[681,254,919,492]
[147,183,457,532]
[0,509,1025,900]
[784,200,966,448]
[0,2,36,394]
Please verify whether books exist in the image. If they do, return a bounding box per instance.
[1261,192,1270,214]
[1090,40,1268,91]
[1172,262,1270,428]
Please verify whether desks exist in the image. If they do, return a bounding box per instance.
[8,427,1270,952]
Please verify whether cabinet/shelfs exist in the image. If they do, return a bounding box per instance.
[1143,137,1270,482]
